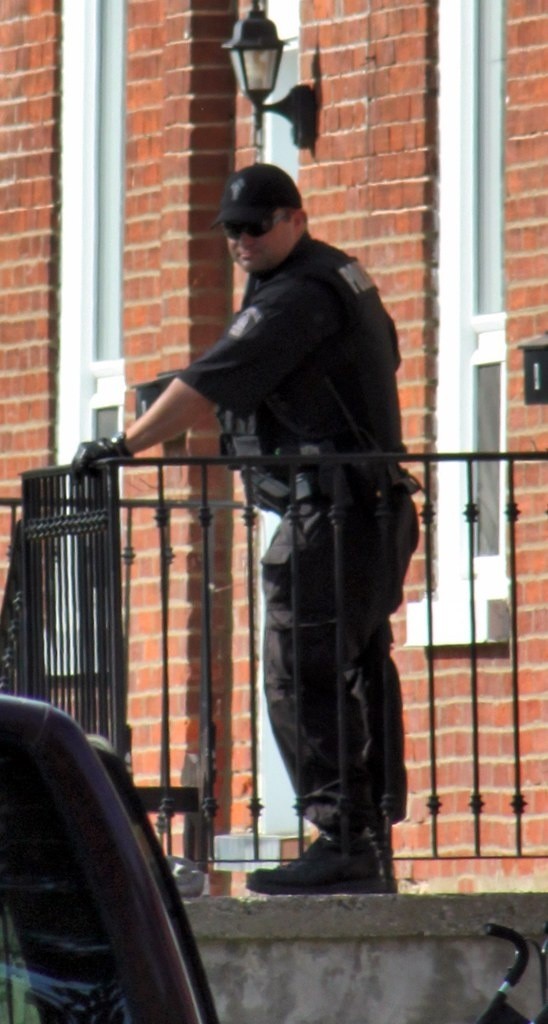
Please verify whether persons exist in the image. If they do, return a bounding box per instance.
[72,161,419,895]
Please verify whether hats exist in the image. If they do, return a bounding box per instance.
[209,162,301,230]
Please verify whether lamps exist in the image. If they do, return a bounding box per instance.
[222,0,316,152]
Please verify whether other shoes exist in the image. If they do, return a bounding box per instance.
[245,836,398,893]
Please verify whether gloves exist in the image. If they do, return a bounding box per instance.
[71,430,133,485]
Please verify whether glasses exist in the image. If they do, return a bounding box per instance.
[222,210,289,239]
